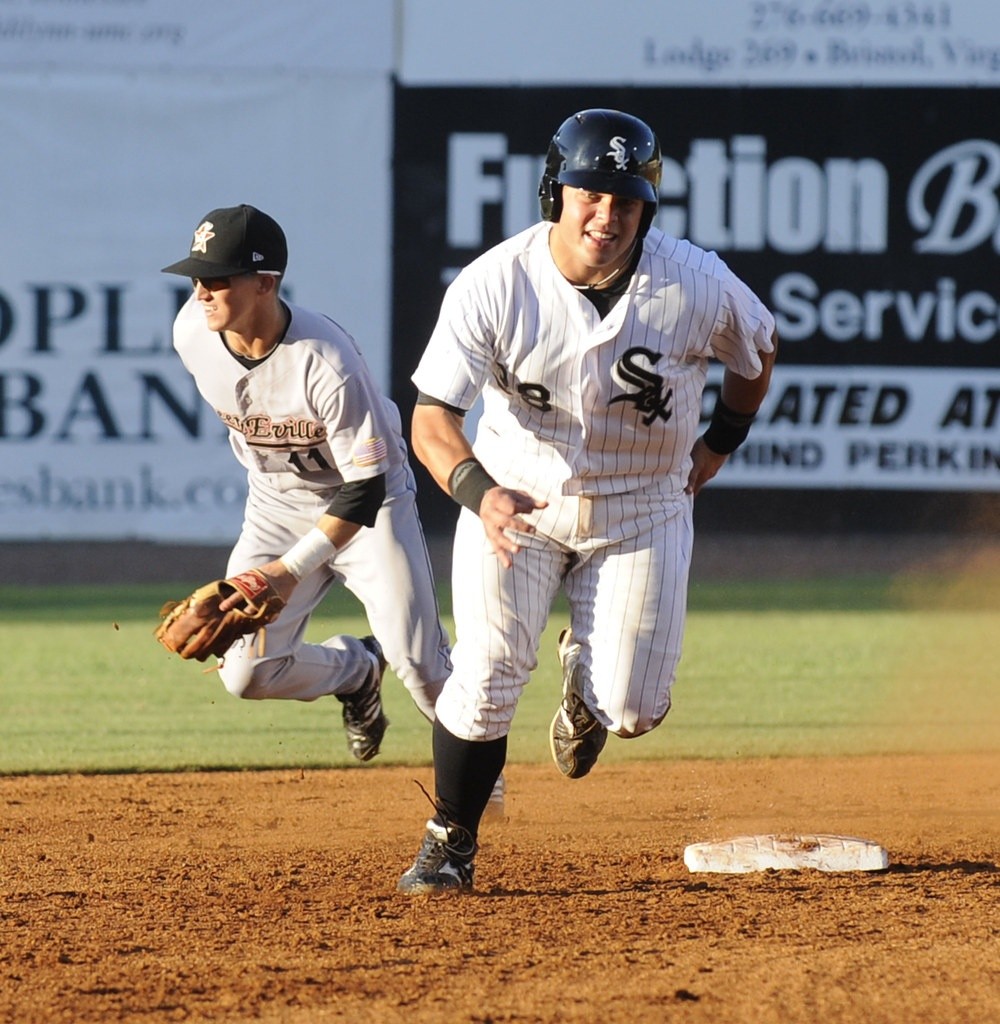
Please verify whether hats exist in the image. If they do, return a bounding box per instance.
[161,203,288,279]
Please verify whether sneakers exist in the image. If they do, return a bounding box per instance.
[395,779,477,896]
[478,773,505,825]
[548,626,609,780]
[334,635,385,761]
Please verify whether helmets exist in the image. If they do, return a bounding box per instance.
[538,109,662,238]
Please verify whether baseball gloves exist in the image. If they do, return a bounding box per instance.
[149,567,287,663]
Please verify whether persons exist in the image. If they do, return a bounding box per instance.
[394,109,777,899]
[156,203,505,816]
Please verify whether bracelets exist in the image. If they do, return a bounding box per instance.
[449,456,498,516]
[279,527,337,586]
[701,394,759,456]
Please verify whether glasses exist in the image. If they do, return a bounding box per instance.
[192,271,266,291]
